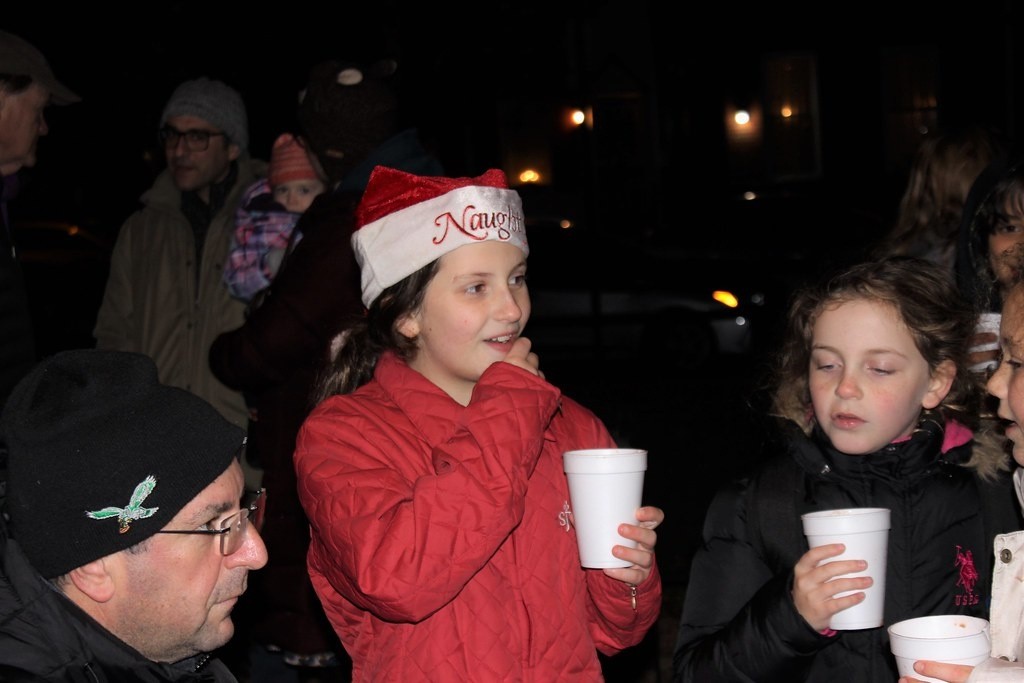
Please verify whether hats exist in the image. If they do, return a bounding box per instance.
[349,165,530,310]
[161,79,248,149]
[270,133,326,185]
[0,349,249,578]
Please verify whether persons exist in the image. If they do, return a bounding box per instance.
[222,133,328,301]
[210,60,480,683]
[0,33,85,399]
[671,121,1024,683]
[0,350,298,683]
[92,77,279,535]
[292,166,664,683]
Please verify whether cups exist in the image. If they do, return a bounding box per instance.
[887,614,992,682]
[562,448,647,569]
[801,507,891,631]
[970,313,1002,376]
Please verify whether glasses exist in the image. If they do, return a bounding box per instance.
[157,125,225,152]
[156,488,268,556]
